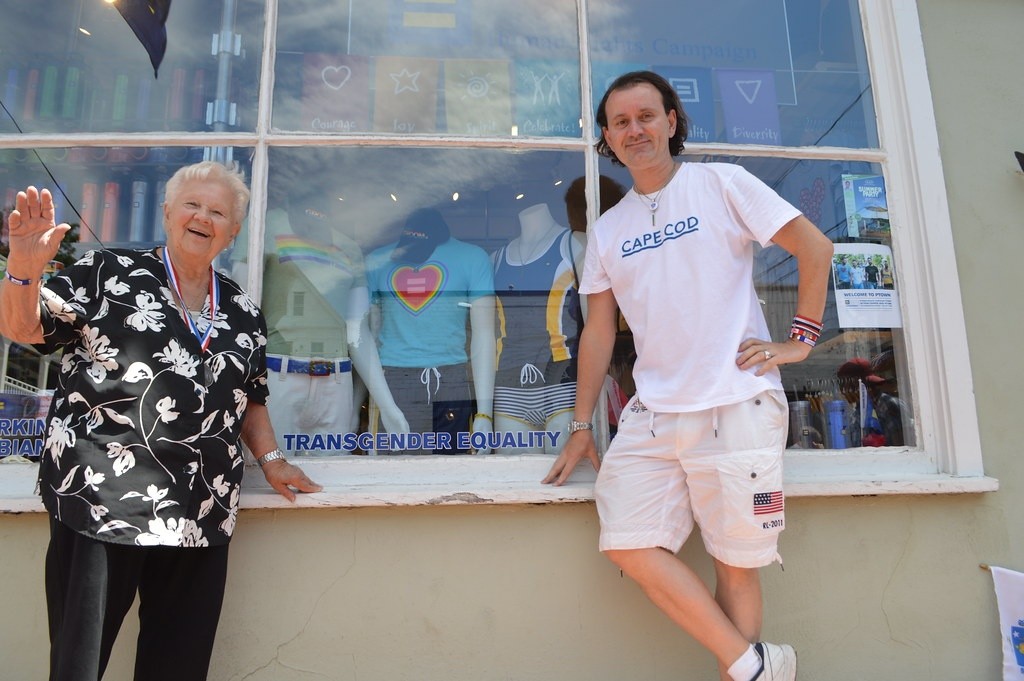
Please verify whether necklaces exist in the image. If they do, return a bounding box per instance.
[162,246,221,353]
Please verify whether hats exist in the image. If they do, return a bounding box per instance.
[837,357,885,385]
[288,182,335,247]
[392,207,451,263]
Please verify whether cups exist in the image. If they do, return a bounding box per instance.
[789,401,813,449]
[37,390,56,419]
[823,400,850,449]
[0,393,39,442]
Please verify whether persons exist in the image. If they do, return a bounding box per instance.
[836,257,893,290]
[267,201,603,456]
[0,160,321,681]
[540,71,835,681]
[844,181,889,230]
[837,359,916,448]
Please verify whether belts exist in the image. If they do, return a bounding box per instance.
[265,355,354,377]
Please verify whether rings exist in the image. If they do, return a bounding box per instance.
[764,351,771,359]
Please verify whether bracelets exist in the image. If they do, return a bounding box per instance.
[475,413,494,423]
[788,313,823,348]
[256,448,286,467]
[5,270,43,287]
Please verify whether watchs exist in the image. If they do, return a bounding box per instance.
[567,419,592,436]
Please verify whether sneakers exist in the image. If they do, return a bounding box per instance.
[749,640,798,681]
